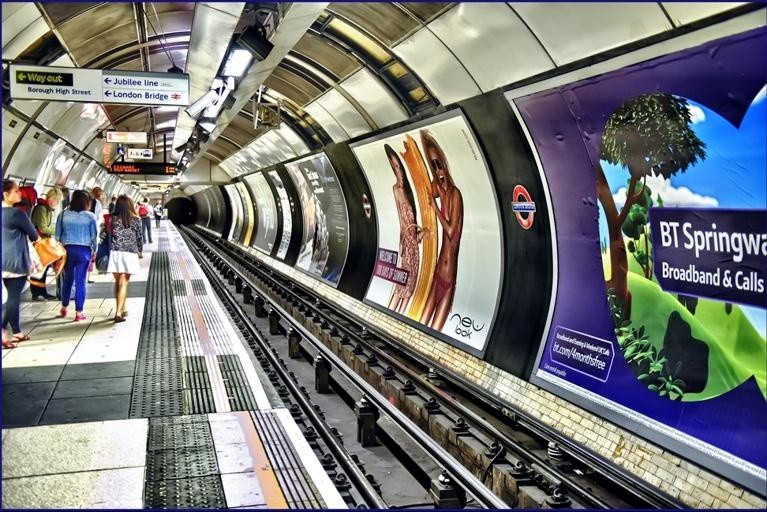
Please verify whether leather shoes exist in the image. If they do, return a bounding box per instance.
[75,313,87,321]
[114,316,126,323]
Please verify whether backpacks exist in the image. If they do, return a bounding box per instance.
[14,186,49,217]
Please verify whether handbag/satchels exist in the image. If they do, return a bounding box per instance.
[95,235,109,275]
[139,205,149,217]
[33,238,65,267]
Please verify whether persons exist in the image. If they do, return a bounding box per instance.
[384,143,423,316]
[408,131,464,333]
[0,179,166,346]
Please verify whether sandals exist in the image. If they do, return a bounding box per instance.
[3,341,17,349]
[12,334,30,343]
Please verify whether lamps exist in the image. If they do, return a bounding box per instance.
[171,7,279,165]
